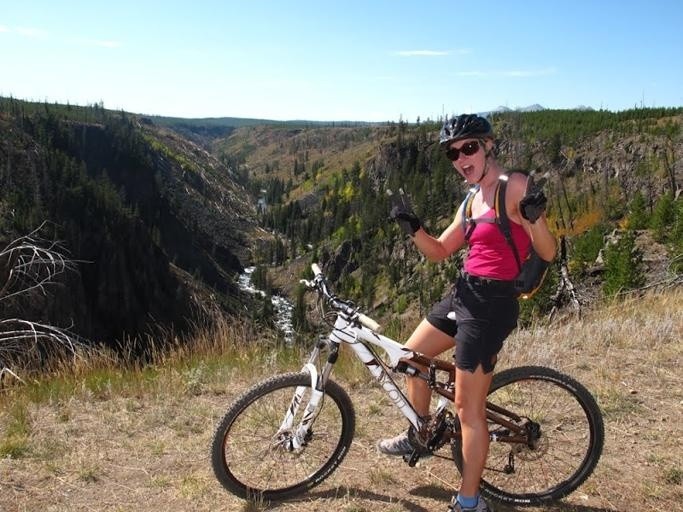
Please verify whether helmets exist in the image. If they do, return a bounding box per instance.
[439,114,493,150]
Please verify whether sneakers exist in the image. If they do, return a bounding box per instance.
[446,491,490,512]
[376,427,414,455]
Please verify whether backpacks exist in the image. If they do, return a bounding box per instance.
[460,169,550,300]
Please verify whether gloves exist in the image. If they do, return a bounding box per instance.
[519,169,550,224]
[386,187,421,242]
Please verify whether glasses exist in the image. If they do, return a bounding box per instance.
[446,141,479,161]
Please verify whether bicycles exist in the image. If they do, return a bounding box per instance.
[212,263,604,505]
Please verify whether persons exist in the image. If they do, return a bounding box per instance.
[379,114,558,512]
